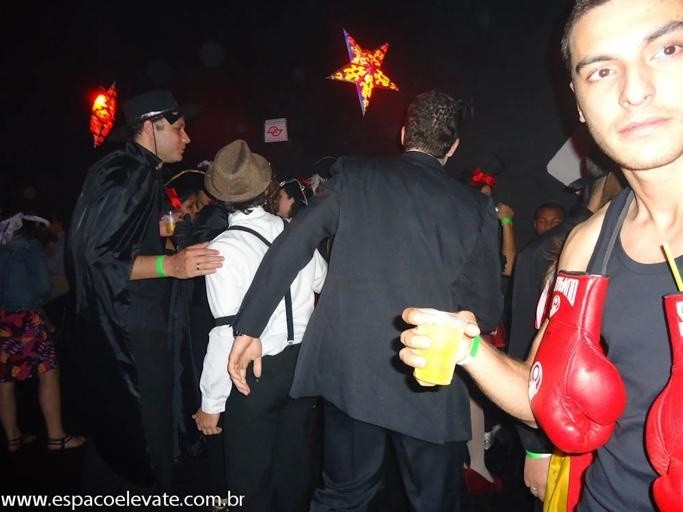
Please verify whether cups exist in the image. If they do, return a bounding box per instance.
[409,309,467,386]
[162,210,178,233]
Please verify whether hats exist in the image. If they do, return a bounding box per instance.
[164,167,209,194]
[104,88,207,144]
[203,139,272,203]
[278,174,309,208]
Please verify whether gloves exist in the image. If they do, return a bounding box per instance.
[645,292,682,509]
[530,265,627,455]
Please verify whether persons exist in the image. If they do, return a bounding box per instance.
[2,88,632,512]
[398,1,683,512]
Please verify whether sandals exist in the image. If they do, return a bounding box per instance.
[7,433,91,457]
